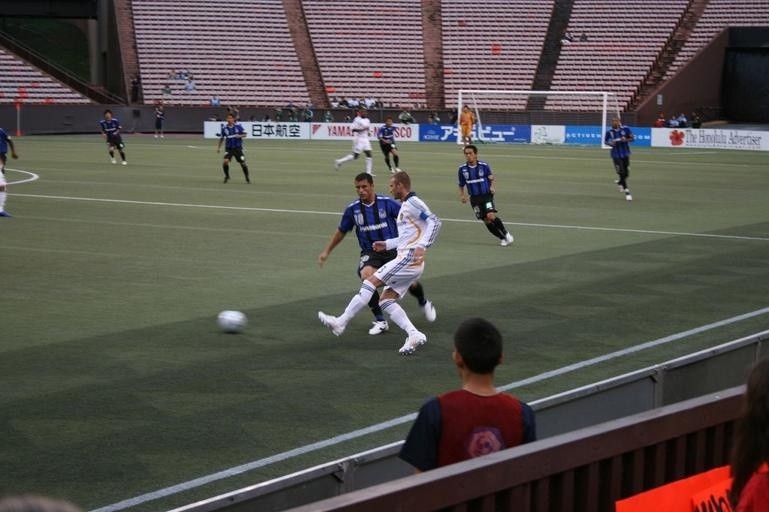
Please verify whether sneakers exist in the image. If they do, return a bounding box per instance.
[318,300,436,355]
[501,239,507,246]
[506,232,513,242]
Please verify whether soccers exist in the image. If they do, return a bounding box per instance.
[219,311,247,333]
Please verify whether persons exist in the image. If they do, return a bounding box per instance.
[1,128,18,217]
[560,31,588,45]
[397,316,539,474]
[250,96,475,177]
[729,359,769,511]
[151,69,249,184]
[605,117,633,201]
[317,169,442,356]
[457,145,514,247]
[316,172,437,335]
[98,109,128,165]
[655,111,702,128]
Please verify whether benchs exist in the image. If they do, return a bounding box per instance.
[0,44,96,105]
